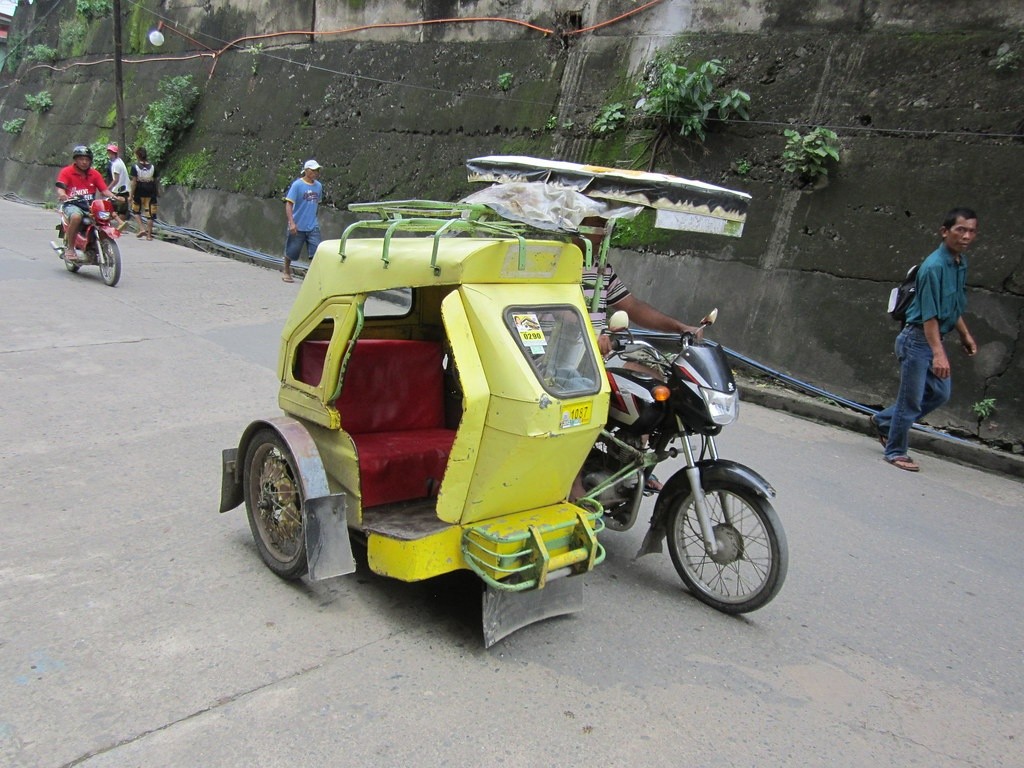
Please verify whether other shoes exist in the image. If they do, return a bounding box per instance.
[121,229,129,234]
[146,236,152,241]
[117,221,128,232]
[136,230,146,237]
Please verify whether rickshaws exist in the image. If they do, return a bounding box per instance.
[217,152,791,650]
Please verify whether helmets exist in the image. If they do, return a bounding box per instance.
[72,146,93,160]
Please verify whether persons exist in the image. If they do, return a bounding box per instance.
[103,145,131,233]
[283,160,323,282]
[869,207,978,471]
[56,146,125,261]
[127,147,160,241]
[572,218,703,494]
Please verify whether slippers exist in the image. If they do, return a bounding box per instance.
[884,453,920,471]
[642,474,662,493]
[869,415,888,448]
[281,276,294,282]
[570,501,596,514]
[65,249,78,260]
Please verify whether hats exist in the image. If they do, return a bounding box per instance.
[107,144,118,152]
[300,160,323,174]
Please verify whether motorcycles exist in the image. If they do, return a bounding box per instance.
[49,183,131,287]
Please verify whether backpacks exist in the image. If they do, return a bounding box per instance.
[887,265,921,320]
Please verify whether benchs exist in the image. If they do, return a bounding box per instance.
[296,340,456,509]
